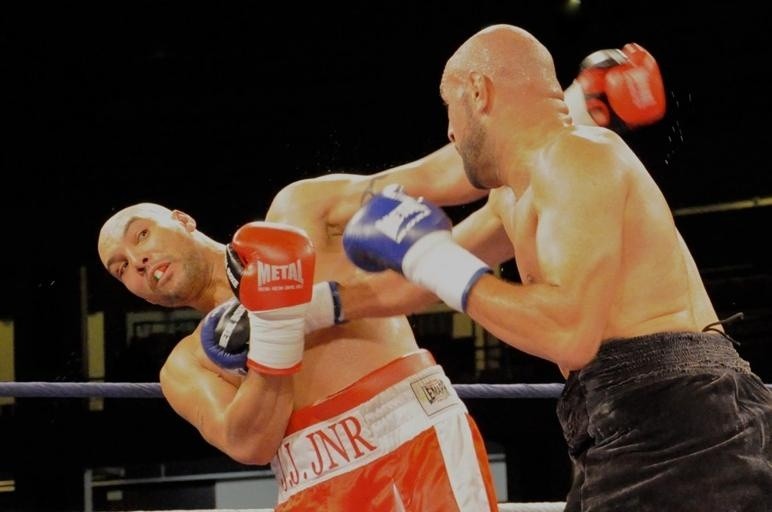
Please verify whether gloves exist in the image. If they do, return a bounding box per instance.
[559,38,668,141]
[198,278,348,374]
[222,218,318,381]
[341,181,494,320]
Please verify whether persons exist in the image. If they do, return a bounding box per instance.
[95,43,667,512]
[197,23,769,510]
